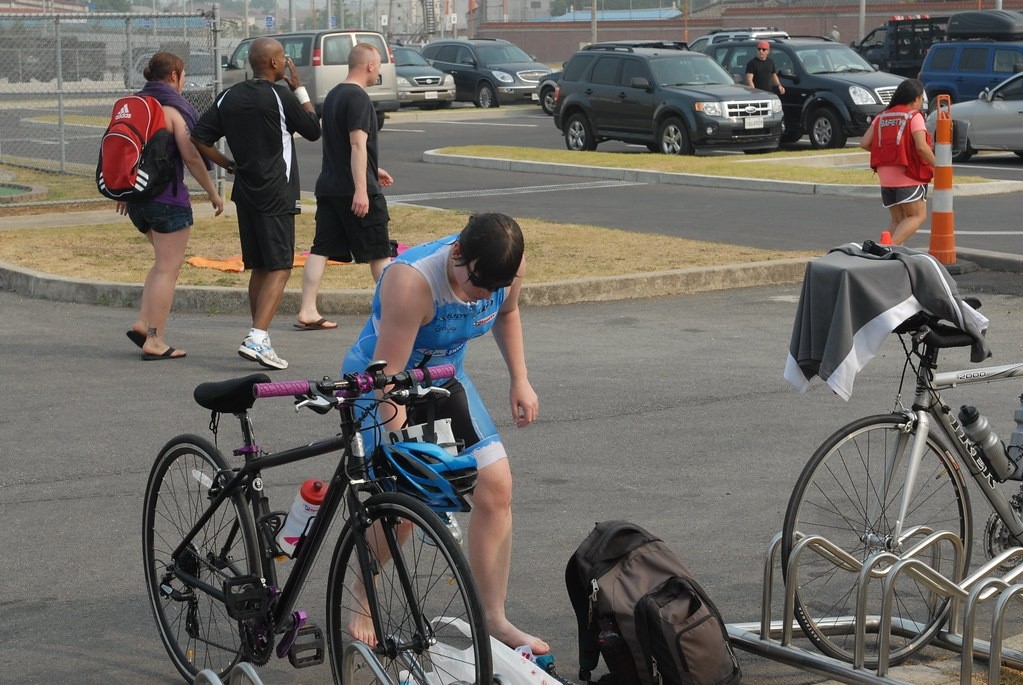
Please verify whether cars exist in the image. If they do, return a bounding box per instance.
[923,70,1023,163]
[378,44,457,112]
[419,38,555,108]
[125,52,225,112]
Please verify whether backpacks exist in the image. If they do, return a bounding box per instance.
[95,94,183,207]
[564,519,743,685]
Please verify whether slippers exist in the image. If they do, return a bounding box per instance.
[293,317,337,330]
[126,330,146,348]
[141,347,186,360]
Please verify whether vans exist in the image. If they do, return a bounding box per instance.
[221,29,400,131]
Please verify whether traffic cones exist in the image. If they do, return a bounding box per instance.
[927,94,958,265]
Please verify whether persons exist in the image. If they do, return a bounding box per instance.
[339,212,550,656]
[292,42,393,330]
[860,79,936,246]
[745,39,785,95]
[830,25,840,41]
[189,37,321,370]
[94,52,223,360]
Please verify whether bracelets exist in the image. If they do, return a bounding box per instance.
[775,83,781,88]
[293,85,311,104]
[227,160,235,174]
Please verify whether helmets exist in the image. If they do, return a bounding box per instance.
[374,442,478,512]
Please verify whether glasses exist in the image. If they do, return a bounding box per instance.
[757,48,767,52]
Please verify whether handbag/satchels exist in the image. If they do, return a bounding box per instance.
[417,616,563,685]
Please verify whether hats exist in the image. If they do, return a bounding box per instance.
[757,40,770,52]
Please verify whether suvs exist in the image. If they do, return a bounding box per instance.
[846,14,969,79]
[536,40,692,116]
[699,34,929,150]
[683,25,792,73]
[553,41,785,156]
[917,10,1023,115]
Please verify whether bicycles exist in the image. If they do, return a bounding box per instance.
[140,349,495,682]
[778,244,1023,670]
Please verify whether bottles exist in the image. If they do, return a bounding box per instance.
[958,393,1023,481]
[276,478,328,557]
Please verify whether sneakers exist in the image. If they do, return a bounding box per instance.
[237,330,289,369]
